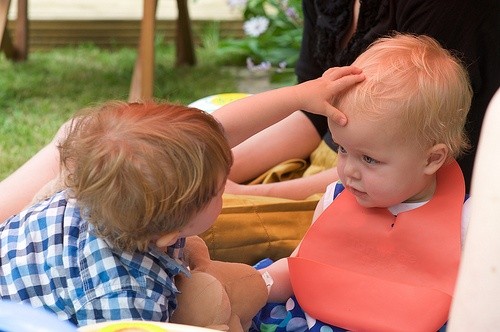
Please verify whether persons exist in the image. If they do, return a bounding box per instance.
[248,28,473,332]
[0,64,367,331]
[1,0,500,266]
